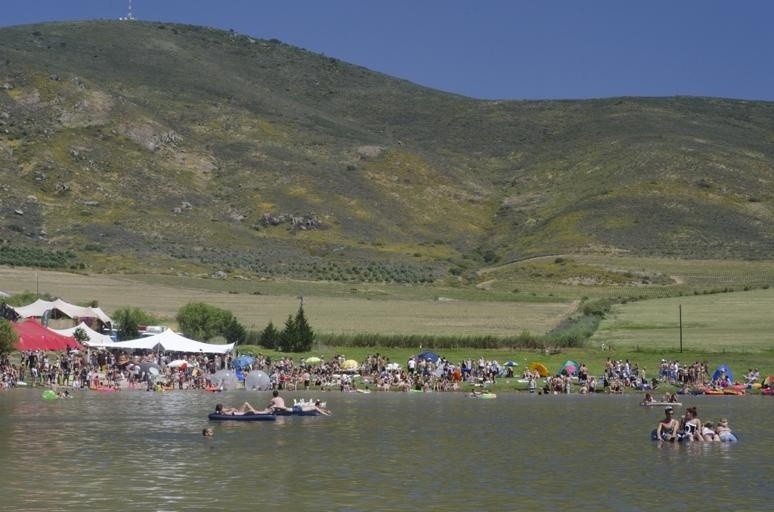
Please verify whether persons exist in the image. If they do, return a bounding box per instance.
[0,344,774,443]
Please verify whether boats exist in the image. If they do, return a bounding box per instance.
[677,388,774,394]
[652,425,737,443]
[408,388,432,394]
[156,386,174,390]
[270,404,322,416]
[91,384,120,392]
[204,386,221,393]
[206,410,274,420]
[352,388,370,394]
[639,401,681,407]
[467,392,496,399]
